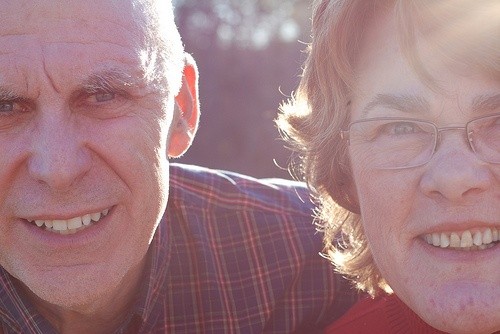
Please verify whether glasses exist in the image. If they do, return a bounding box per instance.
[339,113,500,171]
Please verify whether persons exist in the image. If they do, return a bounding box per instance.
[274,2,500,334]
[0,1,391,333]
